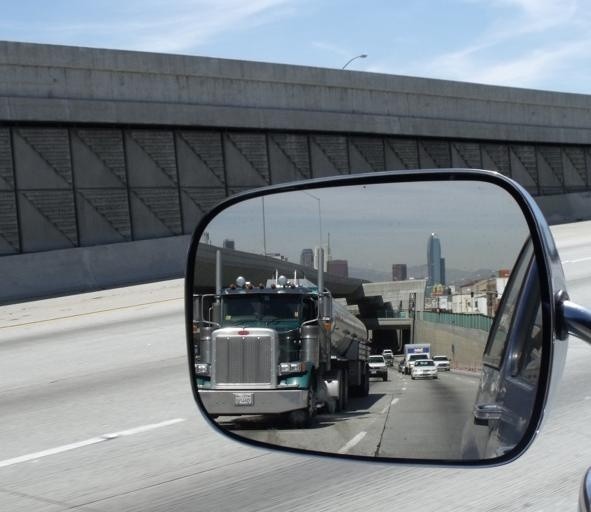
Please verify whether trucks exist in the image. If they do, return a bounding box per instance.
[192,247,372,427]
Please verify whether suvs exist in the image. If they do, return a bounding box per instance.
[367,353,390,382]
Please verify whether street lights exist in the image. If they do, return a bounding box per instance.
[342,51,370,69]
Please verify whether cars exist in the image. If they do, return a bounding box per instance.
[382,342,451,381]
[455,229,541,466]
